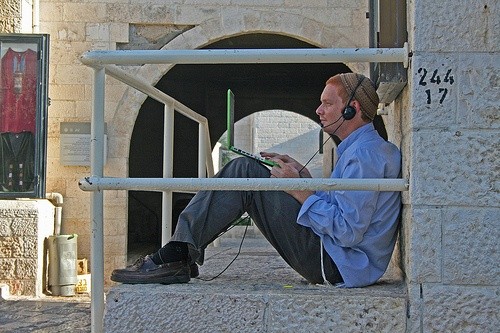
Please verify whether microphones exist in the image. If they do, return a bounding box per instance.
[320,115,343,128]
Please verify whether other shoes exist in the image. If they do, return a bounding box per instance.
[187,258,199,278]
[110,256,190,284]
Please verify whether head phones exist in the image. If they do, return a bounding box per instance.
[342,75,365,120]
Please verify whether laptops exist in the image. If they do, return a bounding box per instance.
[227,89,281,168]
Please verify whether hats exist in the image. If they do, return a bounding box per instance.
[340,73,378,121]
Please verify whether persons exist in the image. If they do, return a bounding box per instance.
[110,72,403,287]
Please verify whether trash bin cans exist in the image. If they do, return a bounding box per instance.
[45,232,79,299]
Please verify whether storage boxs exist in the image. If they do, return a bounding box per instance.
[75,259,91,294]
